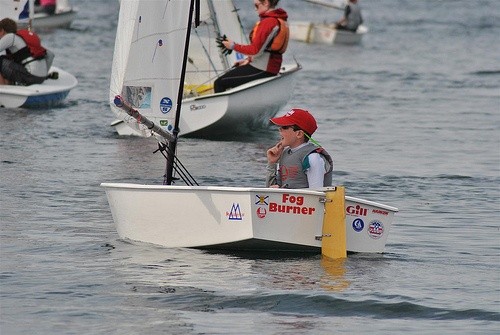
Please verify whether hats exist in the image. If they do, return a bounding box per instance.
[269,108,318,135]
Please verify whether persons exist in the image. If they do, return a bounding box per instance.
[19,0,57,19]
[264,109,333,191]
[322,0,363,33]
[213,0,290,93]
[0,17,48,86]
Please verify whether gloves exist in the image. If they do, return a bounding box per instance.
[216,34,232,56]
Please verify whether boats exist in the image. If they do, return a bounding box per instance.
[285,19,368,45]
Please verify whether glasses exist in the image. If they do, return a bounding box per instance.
[255,2,263,9]
[281,126,300,131]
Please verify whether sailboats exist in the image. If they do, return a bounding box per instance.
[109,0,302,135]
[0,0,78,106]
[99,0,400,258]
[0,0,78,32]
